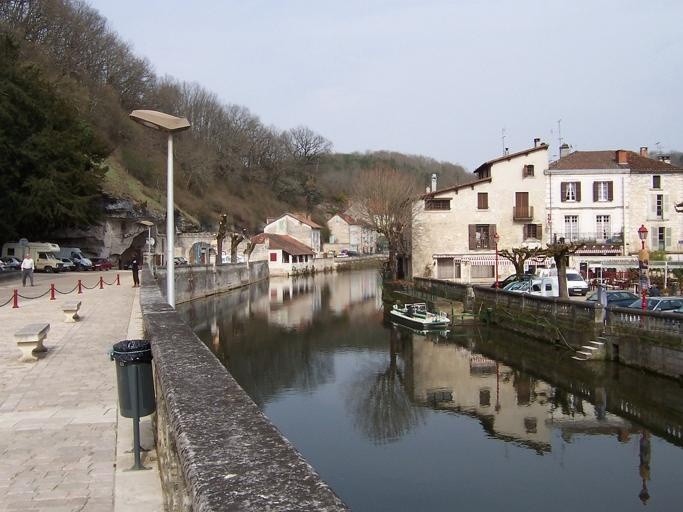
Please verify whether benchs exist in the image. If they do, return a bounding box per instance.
[61,300,82,323]
[14,324,50,362]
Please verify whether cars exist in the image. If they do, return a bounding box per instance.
[0,238,112,273]
[173,256,187,264]
[324,249,357,258]
[625,296,682,314]
[583,290,639,310]
[489,266,588,297]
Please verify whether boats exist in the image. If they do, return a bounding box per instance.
[388,302,451,338]
[389,321,449,345]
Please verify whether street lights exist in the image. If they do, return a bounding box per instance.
[138,220,154,252]
[633,427,652,506]
[492,361,503,415]
[130,109,190,313]
[492,233,499,289]
[637,224,649,328]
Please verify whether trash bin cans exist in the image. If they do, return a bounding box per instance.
[112,340,157,472]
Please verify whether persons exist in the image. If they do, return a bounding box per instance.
[131,256,141,288]
[21,253,34,286]
